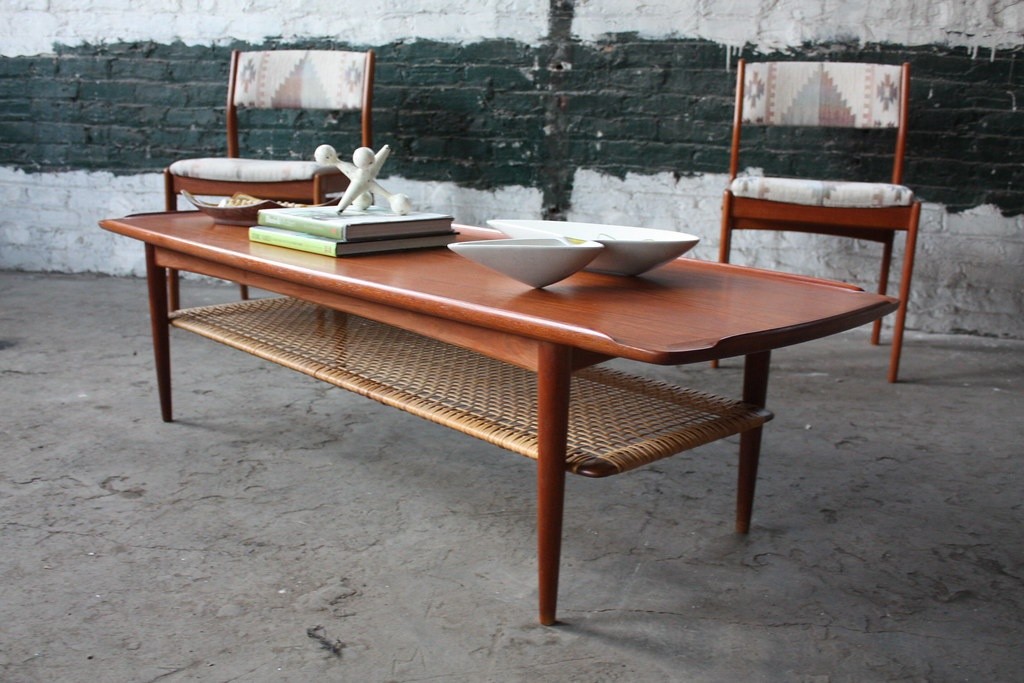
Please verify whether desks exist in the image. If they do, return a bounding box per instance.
[99,209,901,625]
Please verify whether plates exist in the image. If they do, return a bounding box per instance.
[180,190,342,227]
[447,237,604,290]
[487,218,701,277]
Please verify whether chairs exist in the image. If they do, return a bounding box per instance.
[709,58,920,384]
[163,50,375,312]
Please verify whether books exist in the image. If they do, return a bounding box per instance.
[248,203,461,258]
